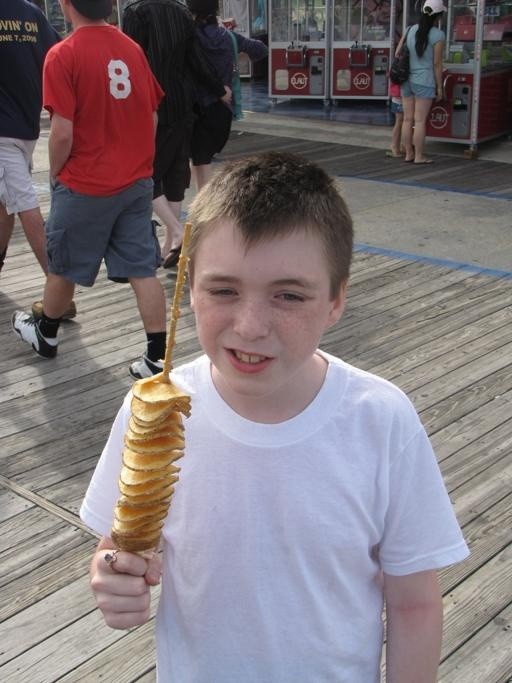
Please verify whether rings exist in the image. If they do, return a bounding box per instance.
[104,550,120,574]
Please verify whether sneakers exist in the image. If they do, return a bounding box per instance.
[128,353,165,381]
[11,301,76,359]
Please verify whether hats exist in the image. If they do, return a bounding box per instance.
[423,0,448,16]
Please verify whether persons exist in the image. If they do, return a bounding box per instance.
[394,0,450,163]
[79,152,471,682]
[386,44,405,156]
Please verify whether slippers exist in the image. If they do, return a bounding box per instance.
[387,152,433,164]
[164,246,181,268]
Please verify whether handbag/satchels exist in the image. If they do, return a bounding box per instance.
[390,26,411,84]
[227,31,242,119]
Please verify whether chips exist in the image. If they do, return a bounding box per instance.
[110,370,192,551]
